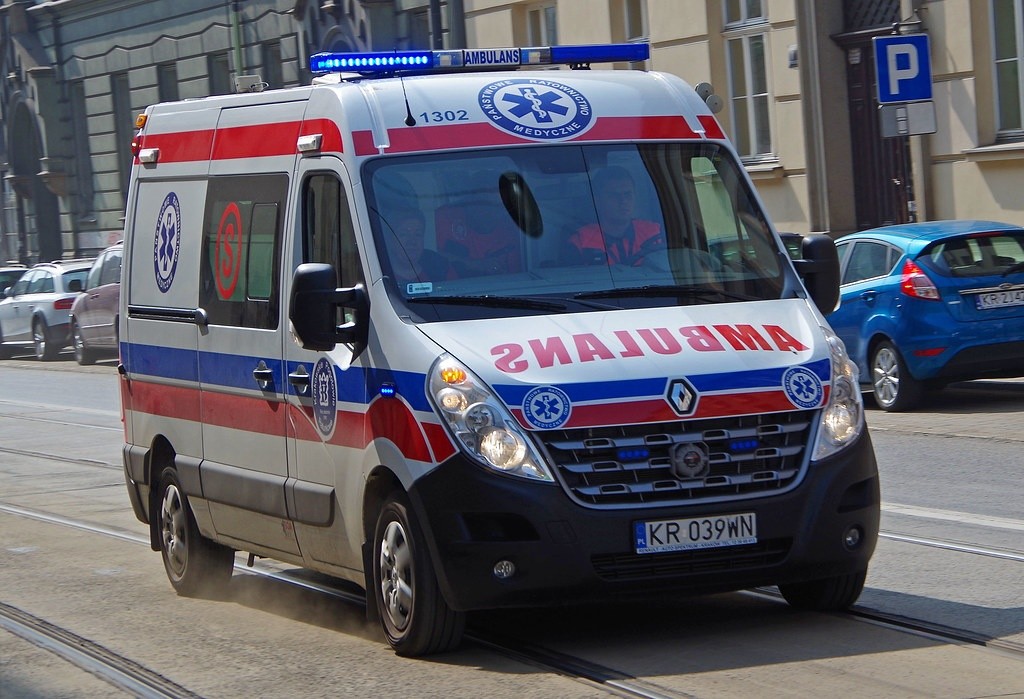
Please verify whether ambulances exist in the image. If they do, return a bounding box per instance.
[121,41,881,654]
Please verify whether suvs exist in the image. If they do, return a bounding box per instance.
[0,256,99,362]
[69,241,122,365]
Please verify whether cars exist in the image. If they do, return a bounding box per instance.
[822,221,1024,412]
[705,233,805,275]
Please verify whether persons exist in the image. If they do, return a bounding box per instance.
[564,166,668,268]
[349,208,458,322]
[436,178,521,277]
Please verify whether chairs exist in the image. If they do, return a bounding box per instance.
[356,171,452,285]
[562,148,668,267]
